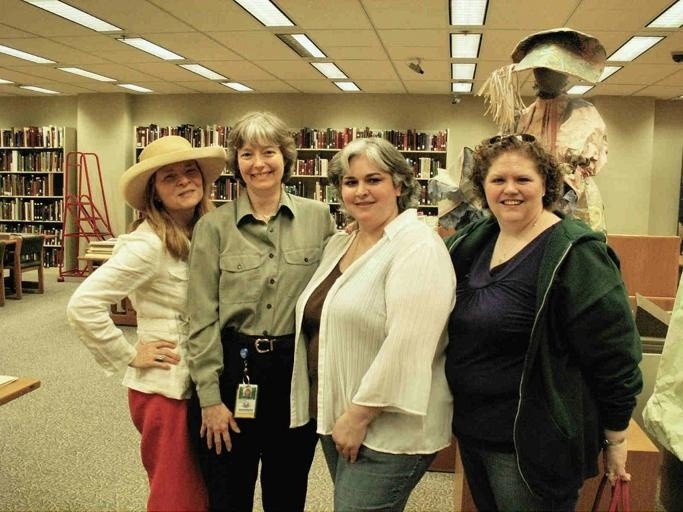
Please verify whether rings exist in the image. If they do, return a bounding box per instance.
[156,355,164,362]
[605,472,615,477]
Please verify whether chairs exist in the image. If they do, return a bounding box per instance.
[1,240,8,308]
[0,233,46,301]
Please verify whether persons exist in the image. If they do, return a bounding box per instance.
[67,134,229,511]
[430,29,608,235]
[288,138,457,511]
[184,112,338,511]
[443,134,644,512]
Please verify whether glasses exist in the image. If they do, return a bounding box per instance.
[488,134,535,145]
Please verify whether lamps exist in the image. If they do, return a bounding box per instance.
[407,56,424,75]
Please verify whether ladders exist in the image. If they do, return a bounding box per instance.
[57,152,115,283]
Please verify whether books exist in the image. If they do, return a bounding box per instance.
[0,124,66,266]
[136,124,243,224]
[84,238,118,260]
[286,125,447,228]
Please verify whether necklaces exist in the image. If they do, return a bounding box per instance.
[498,220,539,264]
[258,210,274,220]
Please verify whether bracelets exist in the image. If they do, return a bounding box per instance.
[605,438,624,448]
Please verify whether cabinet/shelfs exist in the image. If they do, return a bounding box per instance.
[0,126,79,274]
[128,122,452,235]
[75,235,139,328]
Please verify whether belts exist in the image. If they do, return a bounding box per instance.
[226,331,292,352]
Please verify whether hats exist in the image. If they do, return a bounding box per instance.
[122,134,227,214]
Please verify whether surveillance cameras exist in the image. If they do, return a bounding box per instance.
[672,51,683,62]
[451,99,457,105]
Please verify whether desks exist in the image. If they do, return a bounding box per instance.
[1,232,57,309]
